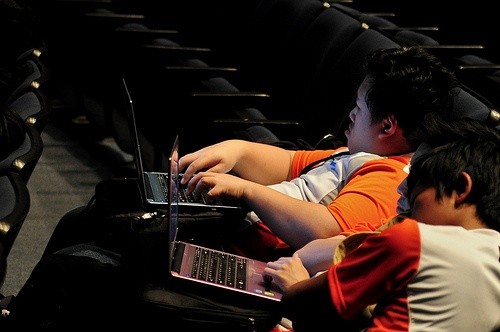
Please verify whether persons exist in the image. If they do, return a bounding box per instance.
[40,46,460,268]
[0,120,499,331]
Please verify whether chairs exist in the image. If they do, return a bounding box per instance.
[0,0,500,287]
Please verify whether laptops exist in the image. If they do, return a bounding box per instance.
[123,77,243,213]
[167,134,284,305]
